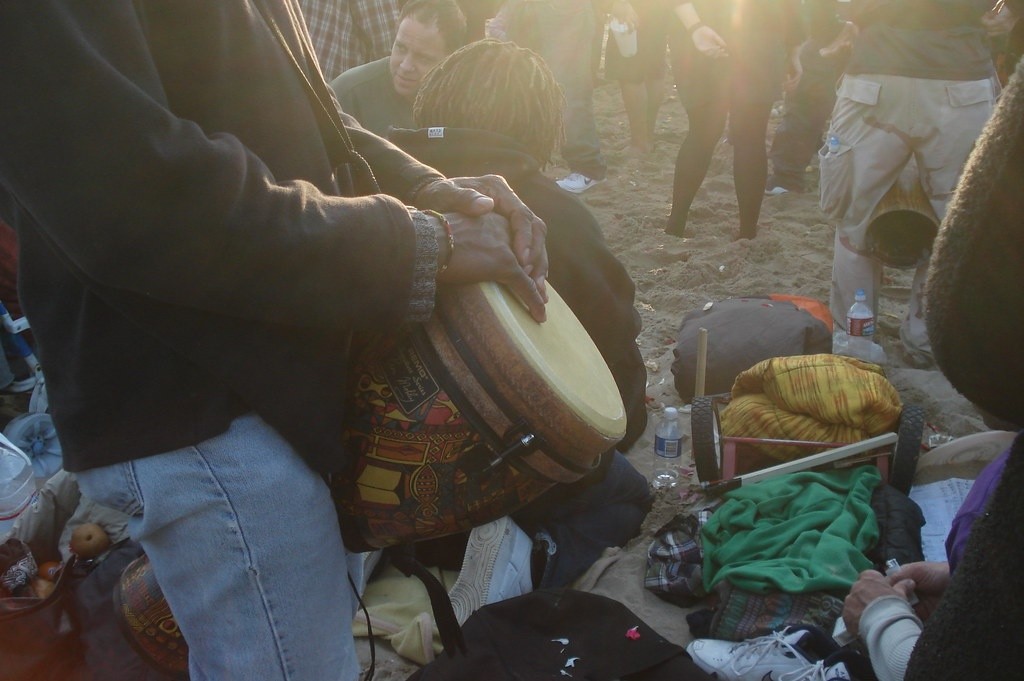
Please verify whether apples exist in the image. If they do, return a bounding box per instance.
[70,523,108,557]
[28,560,60,598]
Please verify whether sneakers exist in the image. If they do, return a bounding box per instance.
[686,624,878,681]
[556,171,607,193]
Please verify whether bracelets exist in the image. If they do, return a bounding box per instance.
[688,22,704,35]
[421,206,455,276]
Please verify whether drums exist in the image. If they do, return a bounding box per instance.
[111,276,631,680]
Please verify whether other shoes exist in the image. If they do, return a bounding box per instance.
[902,346,930,369]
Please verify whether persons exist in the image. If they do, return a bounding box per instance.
[819,0,1024,371]
[297,0,399,84]
[326,0,473,137]
[540,0,1024,681]
[665,0,812,238]
[0,0,551,680]
[407,0,503,38]
[386,38,653,584]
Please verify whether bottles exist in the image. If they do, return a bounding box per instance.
[0,433,38,537]
[823,138,839,158]
[610,18,637,58]
[845,288,875,362]
[652,407,682,490]
